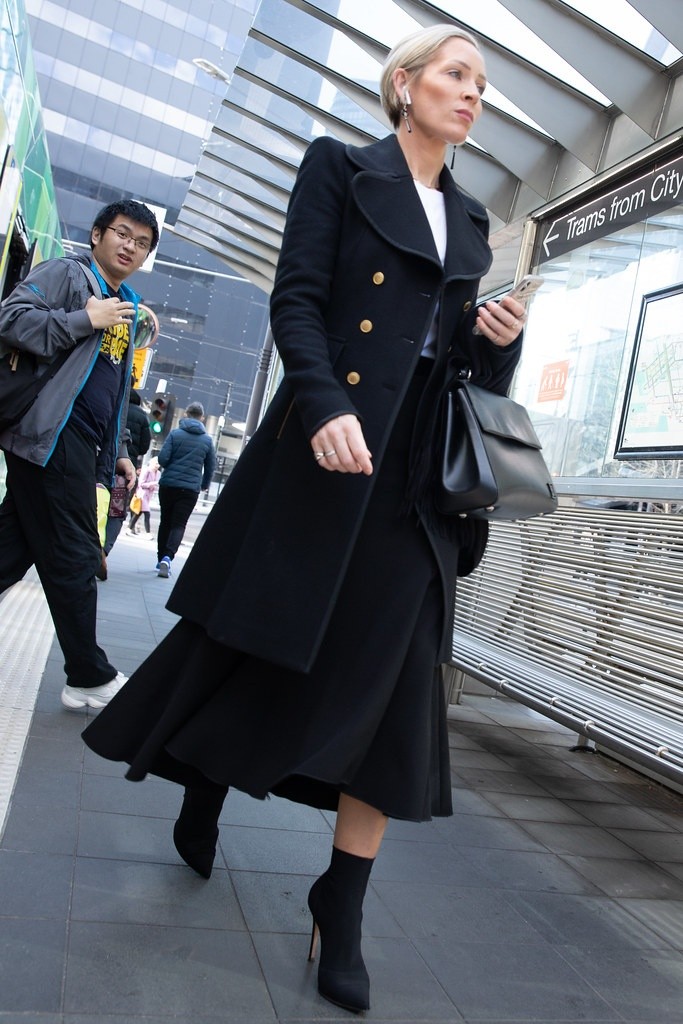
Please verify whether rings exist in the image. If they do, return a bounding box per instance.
[489,310,525,342]
[315,450,336,460]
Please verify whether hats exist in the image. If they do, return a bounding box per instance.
[129,391,141,406]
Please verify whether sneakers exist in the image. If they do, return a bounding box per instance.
[61,670,129,708]
[146,533,154,540]
[126,529,134,537]
[95,548,107,581]
[155,555,172,577]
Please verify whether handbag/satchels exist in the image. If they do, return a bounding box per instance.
[0,257,103,431]
[430,366,558,523]
[129,493,141,516]
[107,487,129,519]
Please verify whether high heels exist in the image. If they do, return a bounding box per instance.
[306,845,377,1014]
[172,766,229,880]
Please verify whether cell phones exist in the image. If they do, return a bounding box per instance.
[471,273,545,336]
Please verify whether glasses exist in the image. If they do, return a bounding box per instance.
[106,225,155,252]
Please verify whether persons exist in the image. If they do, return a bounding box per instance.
[98,378,219,580]
[80,25,530,1014]
[0,200,159,707]
